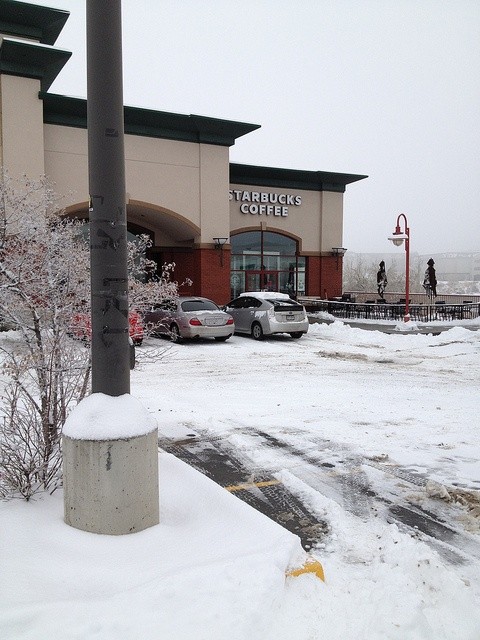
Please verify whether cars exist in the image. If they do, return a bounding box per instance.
[67,292,144,350]
[143,297,236,345]
[221,292,309,340]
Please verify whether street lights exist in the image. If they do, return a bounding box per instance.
[388,213,410,323]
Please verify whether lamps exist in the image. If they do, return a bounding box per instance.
[331,248,344,271]
[213,237,228,268]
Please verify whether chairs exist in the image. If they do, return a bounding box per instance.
[296,291,474,323]
